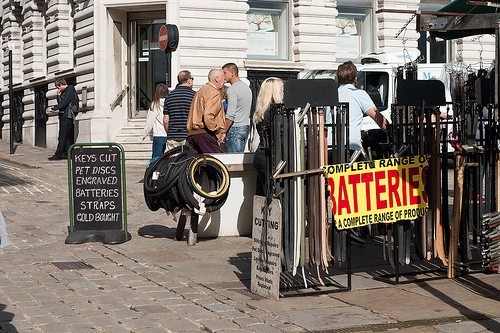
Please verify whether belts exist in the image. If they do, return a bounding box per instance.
[270,103,499,288]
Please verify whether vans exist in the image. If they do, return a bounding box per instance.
[291,63,469,166]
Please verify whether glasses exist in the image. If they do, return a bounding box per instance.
[186,78,194,80]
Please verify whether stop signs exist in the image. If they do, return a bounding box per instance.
[157,25,169,51]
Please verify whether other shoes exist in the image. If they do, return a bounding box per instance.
[48,155,61,160]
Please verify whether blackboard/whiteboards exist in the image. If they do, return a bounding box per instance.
[71,147,123,230]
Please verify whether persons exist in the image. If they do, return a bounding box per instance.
[219,62,253,154]
[187,69,226,156]
[320,61,389,244]
[254,77,285,200]
[464,103,490,205]
[45,78,80,161]
[142,84,170,161]
[163,70,197,157]
